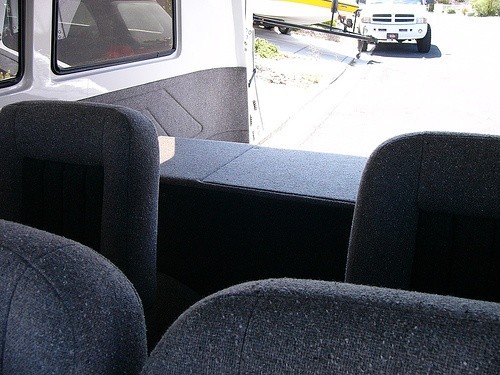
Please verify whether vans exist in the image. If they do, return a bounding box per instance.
[356,1,433,55]
[0,0,262,168]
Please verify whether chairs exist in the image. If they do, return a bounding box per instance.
[0,101,161,374]
[139,131,500,375]
